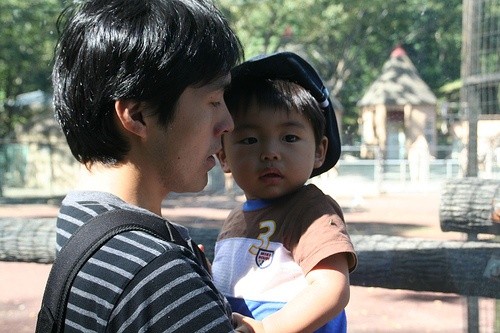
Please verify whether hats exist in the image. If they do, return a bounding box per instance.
[223,52,341,178]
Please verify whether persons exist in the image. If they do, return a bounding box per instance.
[53,0,246,333]
[211,51,359,333]
[406,120,500,192]
